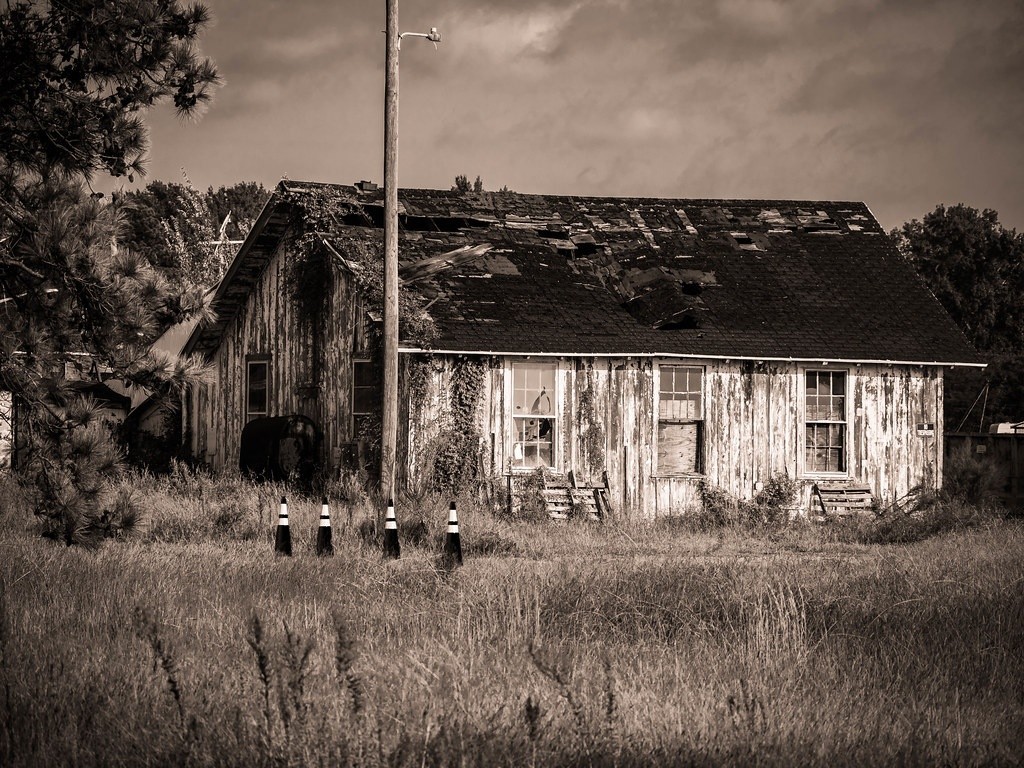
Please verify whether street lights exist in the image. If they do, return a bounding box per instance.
[381,23,443,505]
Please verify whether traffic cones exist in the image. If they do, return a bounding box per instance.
[271,495,296,557]
[440,501,465,561]
[312,497,337,559]
[383,499,406,561]
